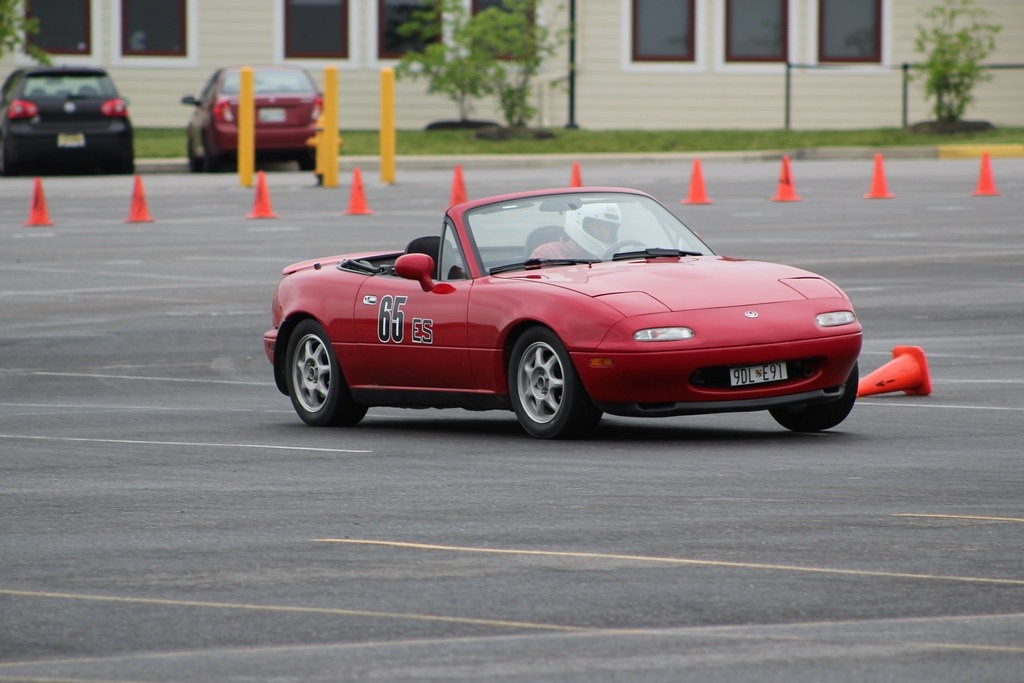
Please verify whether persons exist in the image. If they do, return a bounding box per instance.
[529,198,622,263]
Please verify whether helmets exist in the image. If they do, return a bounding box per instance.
[566,198,624,259]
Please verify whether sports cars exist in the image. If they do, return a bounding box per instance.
[261,179,865,442]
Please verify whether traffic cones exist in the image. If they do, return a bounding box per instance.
[22,177,53,226]
[568,161,584,188]
[856,346,934,397]
[679,158,716,205]
[246,169,277,219]
[862,153,896,200]
[341,167,376,216]
[447,165,468,209]
[124,174,154,222]
[970,148,1001,196]
[767,154,803,203]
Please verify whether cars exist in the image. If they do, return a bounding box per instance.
[181,62,323,174]
[0,66,135,175]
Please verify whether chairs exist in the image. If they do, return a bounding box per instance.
[523,226,565,263]
[403,236,457,280]
